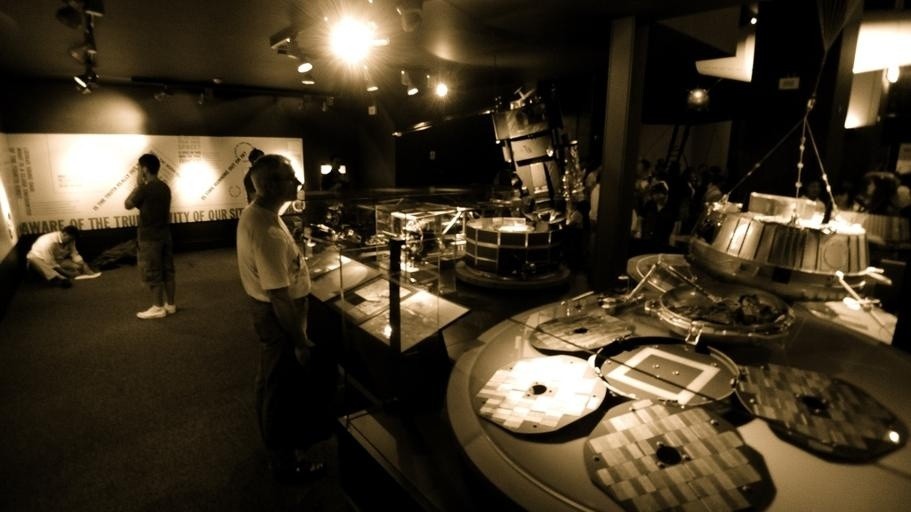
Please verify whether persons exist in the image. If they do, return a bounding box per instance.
[126,154,177,319]
[243,149,269,211]
[27,227,96,287]
[238,155,311,480]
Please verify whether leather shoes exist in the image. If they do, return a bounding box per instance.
[296,462,324,474]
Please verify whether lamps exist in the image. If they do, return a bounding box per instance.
[271,25,316,88]
[69,0,107,96]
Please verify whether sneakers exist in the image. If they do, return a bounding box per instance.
[136,303,176,320]
[60,277,72,288]
